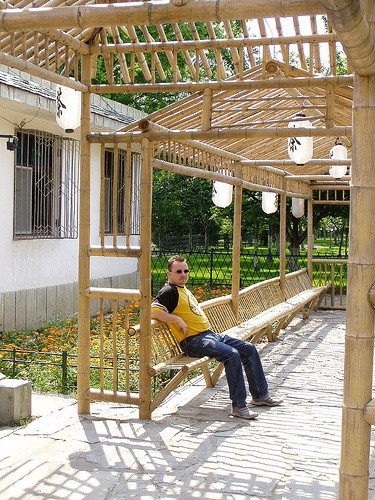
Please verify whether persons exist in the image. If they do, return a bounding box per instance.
[150,255,283,419]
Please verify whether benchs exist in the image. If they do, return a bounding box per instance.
[285,268,332,320]
[230,277,300,343]
[127,296,256,414]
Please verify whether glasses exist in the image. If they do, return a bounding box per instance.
[172,269,189,274]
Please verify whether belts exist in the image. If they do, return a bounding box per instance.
[180,329,209,347]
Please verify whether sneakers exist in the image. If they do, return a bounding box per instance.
[232,406,258,419]
[252,393,283,406]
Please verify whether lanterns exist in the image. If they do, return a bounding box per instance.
[212,169,232,208]
[55,74,80,133]
[329,143,347,179]
[261,191,279,214]
[292,198,304,218]
[288,113,313,165]
